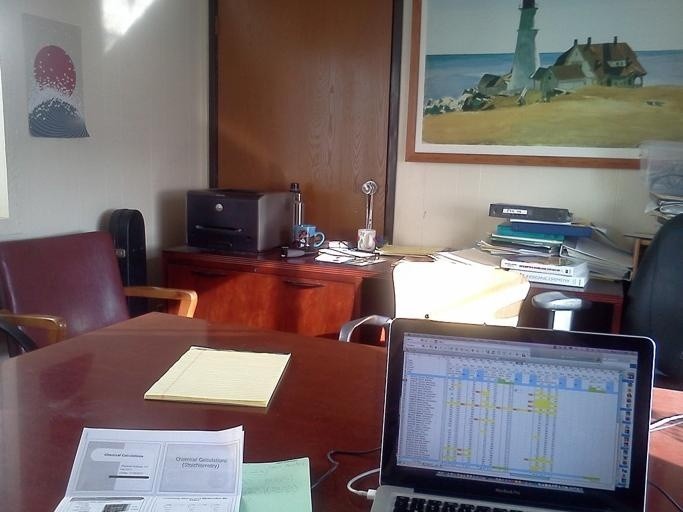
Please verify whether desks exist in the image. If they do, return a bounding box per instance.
[0,309,683,512]
[160,241,627,338]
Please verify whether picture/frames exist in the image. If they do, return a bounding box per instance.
[405,0,682,170]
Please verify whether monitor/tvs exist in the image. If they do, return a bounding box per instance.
[368,317,657,512]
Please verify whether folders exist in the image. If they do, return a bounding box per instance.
[513,223,592,238]
[490,205,573,223]
[498,224,568,239]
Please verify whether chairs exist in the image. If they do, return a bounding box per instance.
[628,212,683,388]
[0,230,198,357]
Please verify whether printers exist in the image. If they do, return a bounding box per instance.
[185,188,293,254]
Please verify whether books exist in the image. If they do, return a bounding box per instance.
[500,255,591,289]
[144,344,291,407]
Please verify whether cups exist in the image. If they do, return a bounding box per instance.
[293,224,324,251]
[357,228,376,252]
